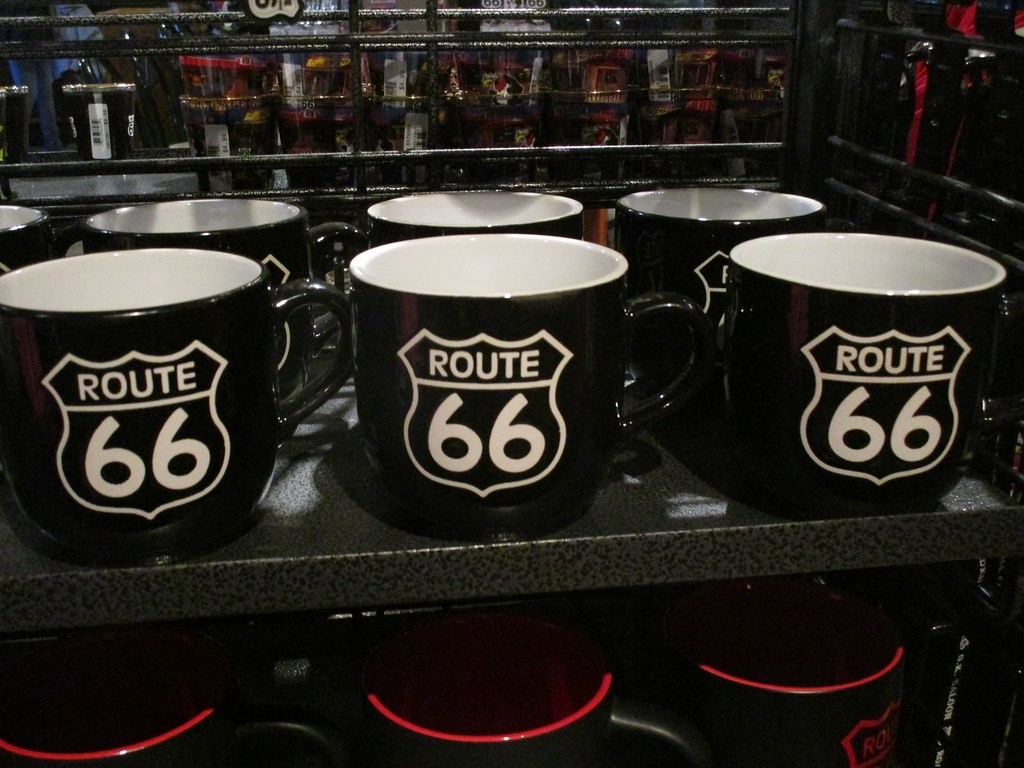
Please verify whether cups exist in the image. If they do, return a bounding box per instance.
[1,190,1008,567]
[1,572,1022,767]
